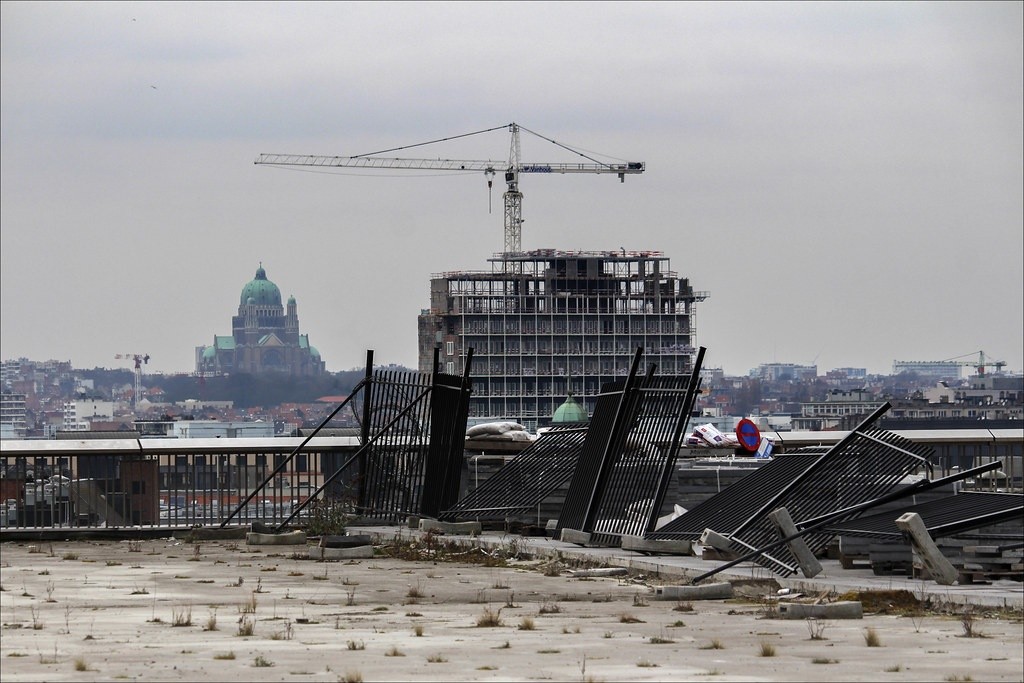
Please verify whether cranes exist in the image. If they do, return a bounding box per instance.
[892,350,1006,375]
[115,354,150,406]
[254,121,646,274]
